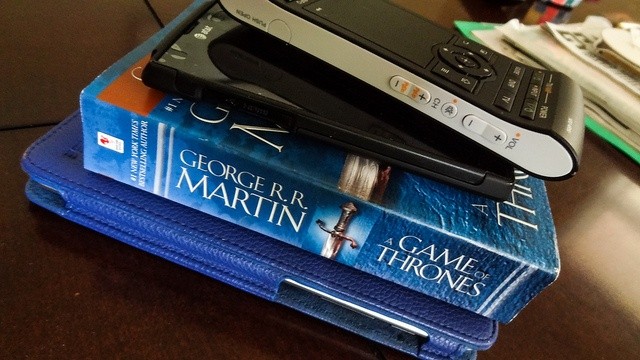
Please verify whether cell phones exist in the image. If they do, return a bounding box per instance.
[141,0,515,202]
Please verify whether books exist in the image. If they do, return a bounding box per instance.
[80,2,560,323]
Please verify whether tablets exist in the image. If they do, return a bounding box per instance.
[283,277,428,338]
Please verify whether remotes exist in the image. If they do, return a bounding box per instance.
[218,1,584,181]
